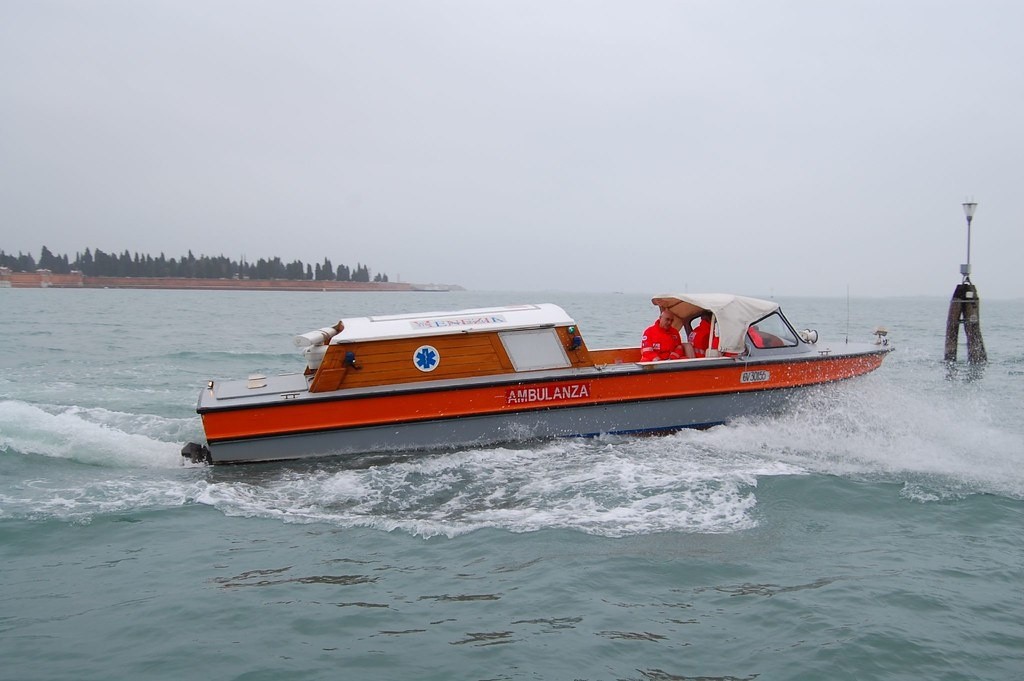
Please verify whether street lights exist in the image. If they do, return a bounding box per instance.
[959,200,978,284]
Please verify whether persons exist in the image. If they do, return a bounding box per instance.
[640,310,688,362]
[690,309,765,358]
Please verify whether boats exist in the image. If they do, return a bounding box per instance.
[177,290,899,469]
